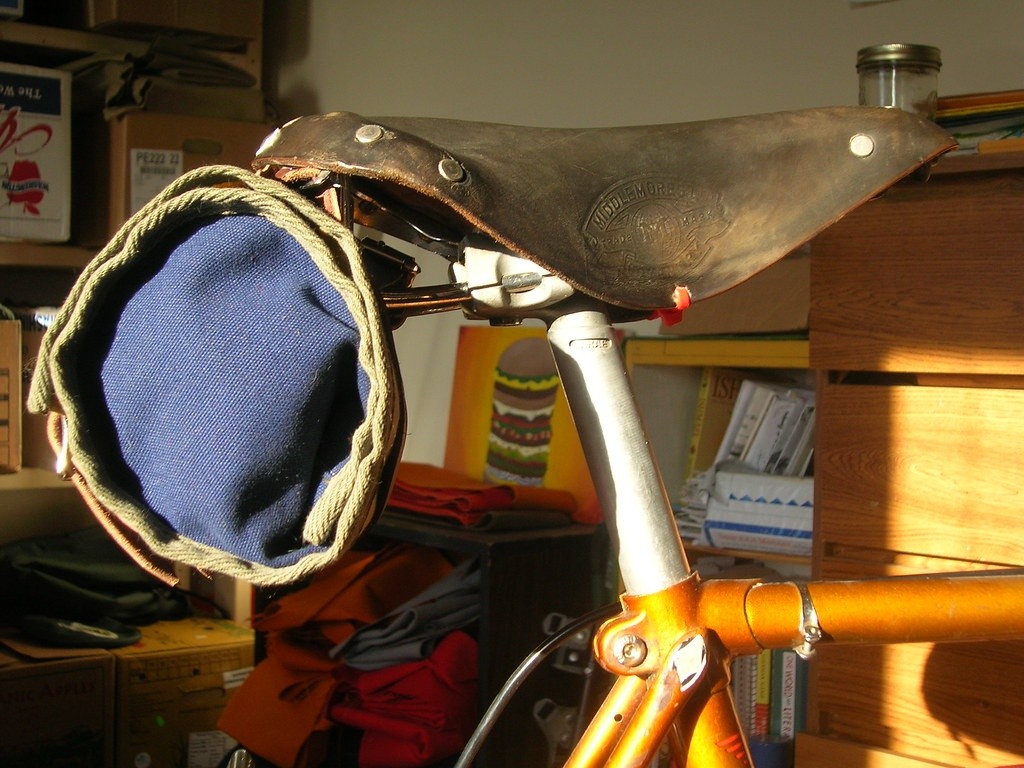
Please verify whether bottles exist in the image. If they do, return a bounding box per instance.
[856,43,943,181]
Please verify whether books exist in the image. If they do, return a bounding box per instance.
[936,89,1024,155]
[675,366,816,556]
[730,648,806,740]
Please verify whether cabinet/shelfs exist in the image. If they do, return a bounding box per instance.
[247,514,598,768]
[796,151,1024,768]
[0,0,264,768]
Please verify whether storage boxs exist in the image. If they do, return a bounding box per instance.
[0,61,76,245]
[81,0,259,47]
[695,469,816,560]
[112,615,254,768]
[651,254,809,334]
[0,0,26,22]
[0,320,23,478]
[80,109,278,250]
[0,634,116,768]
[10,306,63,474]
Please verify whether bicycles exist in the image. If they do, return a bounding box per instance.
[251,105,1024,768]
